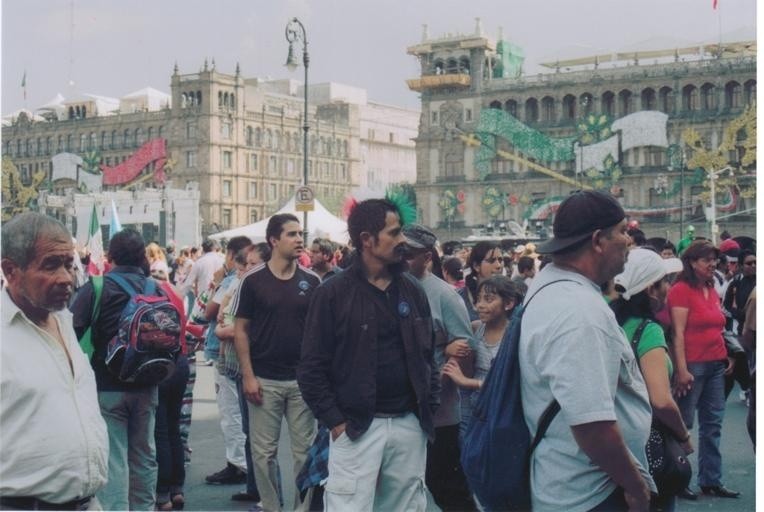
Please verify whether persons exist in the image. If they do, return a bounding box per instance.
[517,187,662,512]
[400,225,553,508]
[599,225,755,508]
[234,215,323,510]
[234,212,323,510]
[66,229,272,508]
[1,211,110,511]
[295,196,436,511]
[296,238,358,283]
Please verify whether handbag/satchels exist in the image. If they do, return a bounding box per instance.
[646,416,692,496]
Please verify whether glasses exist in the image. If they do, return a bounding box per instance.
[701,257,721,264]
[743,260,756,266]
[403,249,429,260]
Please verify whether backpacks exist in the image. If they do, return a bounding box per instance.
[90,272,182,385]
[460,278,584,512]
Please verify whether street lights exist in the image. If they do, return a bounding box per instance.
[283,18,312,249]
[706,165,734,246]
[667,143,684,243]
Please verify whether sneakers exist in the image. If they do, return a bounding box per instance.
[206,465,247,485]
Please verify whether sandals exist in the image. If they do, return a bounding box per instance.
[155,502,173,512]
[171,492,185,510]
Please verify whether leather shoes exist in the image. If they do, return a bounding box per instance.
[232,490,261,502]
[677,487,697,499]
[701,484,739,497]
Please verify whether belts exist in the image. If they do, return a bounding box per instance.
[0,496,91,510]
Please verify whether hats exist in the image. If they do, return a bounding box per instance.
[400,222,437,250]
[536,189,624,254]
[614,248,684,301]
